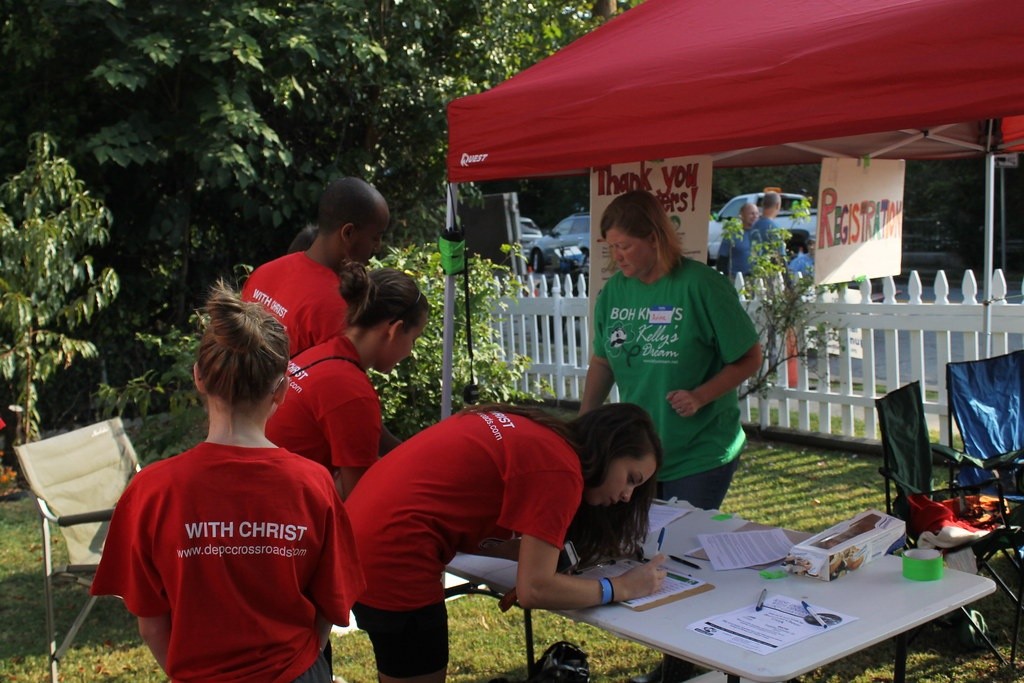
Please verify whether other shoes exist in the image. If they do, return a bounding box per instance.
[630,656,694,683]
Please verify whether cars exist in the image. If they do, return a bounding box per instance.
[896,213,968,282]
[517,212,590,276]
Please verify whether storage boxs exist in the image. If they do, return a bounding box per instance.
[790,508,906,582]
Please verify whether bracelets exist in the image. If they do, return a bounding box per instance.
[598,576,614,606]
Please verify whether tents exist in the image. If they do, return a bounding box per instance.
[441,0,1024,418]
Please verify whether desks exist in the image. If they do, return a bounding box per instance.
[444,496,998,683]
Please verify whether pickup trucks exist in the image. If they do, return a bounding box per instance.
[706,193,818,265]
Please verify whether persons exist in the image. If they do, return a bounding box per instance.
[717,201,762,278]
[577,191,763,510]
[264,269,433,503]
[239,177,390,360]
[753,192,787,257]
[90,280,369,683]
[787,239,813,277]
[342,402,668,683]
[288,223,319,254]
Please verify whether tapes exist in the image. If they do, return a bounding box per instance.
[902,547,943,581]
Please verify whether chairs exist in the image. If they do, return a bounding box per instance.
[875,349,1024,671]
[13,413,144,683]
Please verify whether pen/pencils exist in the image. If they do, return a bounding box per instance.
[655,527,665,555]
[801,601,828,629]
[756,589,767,611]
[667,554,703,569]
